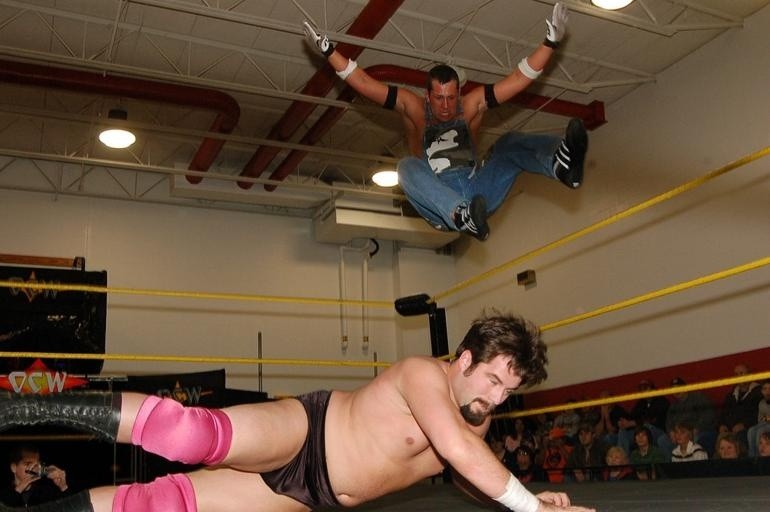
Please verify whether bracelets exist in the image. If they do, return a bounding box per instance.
[336,59,359,79]
[492,473,539,512]
[515,56,544,79]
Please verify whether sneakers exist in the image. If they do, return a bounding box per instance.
[454,194,490,242]
[551,119,588,189]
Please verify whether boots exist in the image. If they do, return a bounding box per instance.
[0,391,122,448]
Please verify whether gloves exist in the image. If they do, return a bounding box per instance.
[303,22,334,57]
[542,1,569,49]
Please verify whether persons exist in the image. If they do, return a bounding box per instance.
[487,363,770,483]
[303,1,588,242]
[0,443,68,508]
[0,308,595,512]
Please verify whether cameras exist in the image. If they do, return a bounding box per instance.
[41,465,49,476]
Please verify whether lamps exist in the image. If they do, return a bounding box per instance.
[371,153,400,187]
[97,109,137,148]
[590,0,635,11]
